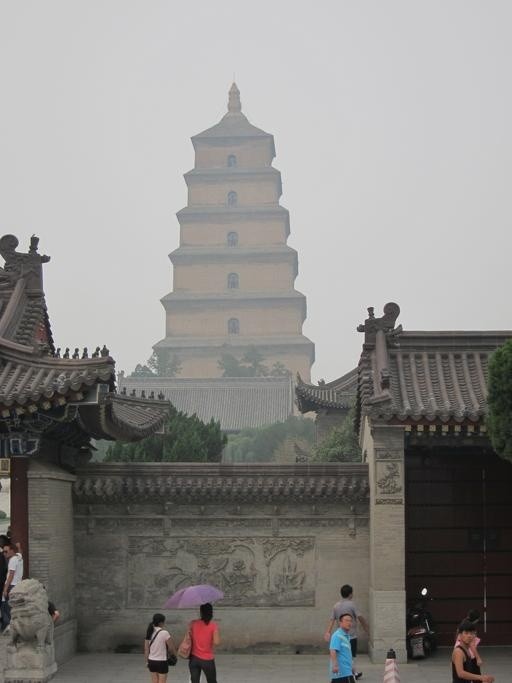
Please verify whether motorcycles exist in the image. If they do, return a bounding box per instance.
[406,587,436,659]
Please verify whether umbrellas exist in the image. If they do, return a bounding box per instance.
[164,584,225,621]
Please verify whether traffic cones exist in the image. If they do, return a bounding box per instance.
[383,648,400,683]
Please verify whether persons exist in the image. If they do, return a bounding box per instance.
[145,613,176,683]
[325,584,370,682]
[451,609,496,683]
[188,602,220,683]
[0,534,24,633]
[48,602,60,623]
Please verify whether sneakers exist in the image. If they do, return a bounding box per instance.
[354,670,362,681]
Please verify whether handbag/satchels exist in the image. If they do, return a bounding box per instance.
[166,653,178,667]
[177,630,192,661]
